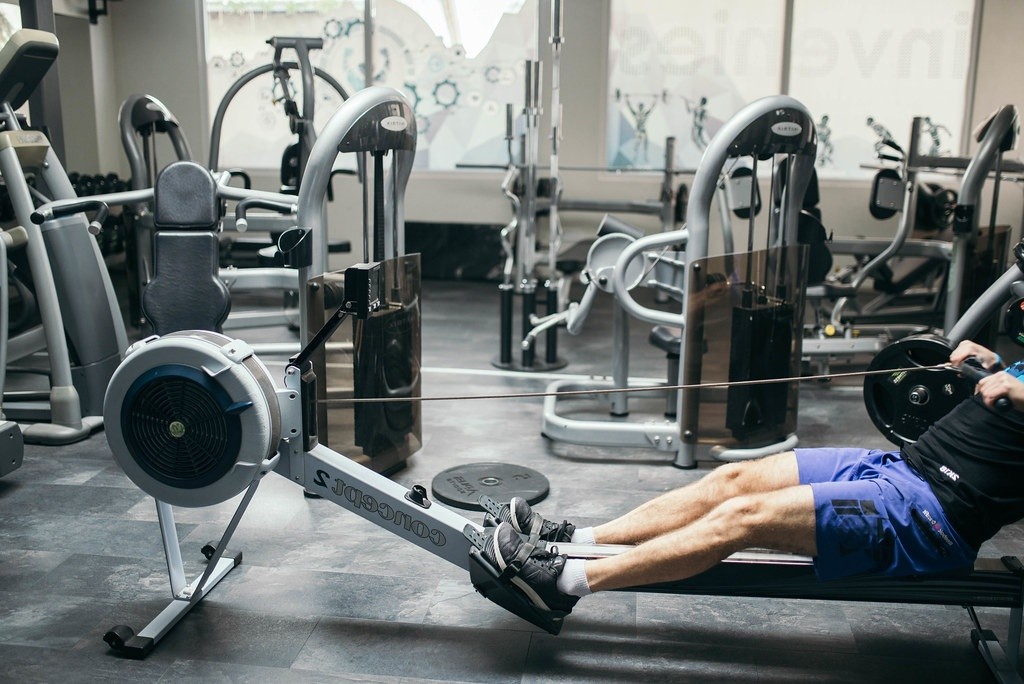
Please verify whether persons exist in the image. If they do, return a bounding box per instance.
[493,340,1024,621]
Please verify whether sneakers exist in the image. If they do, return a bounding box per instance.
[510,497,575,543]
[494,522,581,621]
